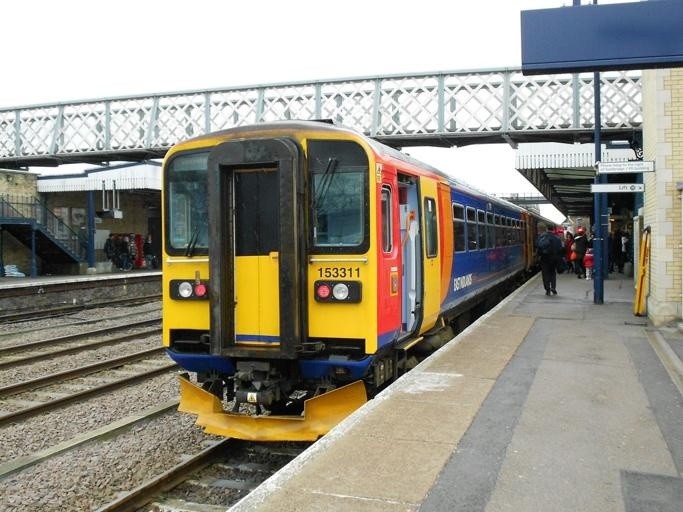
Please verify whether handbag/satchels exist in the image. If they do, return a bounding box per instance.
[557,259,569,274]
[570,242,577,251]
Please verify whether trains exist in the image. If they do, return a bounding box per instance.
[160,118,563,443]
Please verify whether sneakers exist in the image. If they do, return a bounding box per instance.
[578,276,592,280]
[551,288,557,294]
[546,290,550,295]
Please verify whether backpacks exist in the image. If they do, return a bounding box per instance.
[538,236,553,256]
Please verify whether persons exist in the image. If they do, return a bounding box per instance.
[535,222,632,281]
[103,231,160,269]
[78,222,87,261]
[534,222,564,295]
